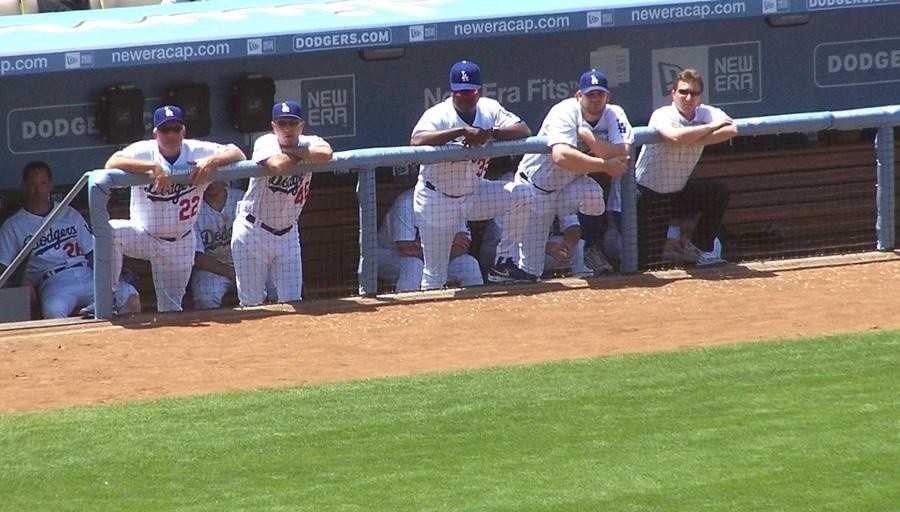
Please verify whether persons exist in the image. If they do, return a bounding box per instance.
[634,68,737,268]
[514,64,636,279]
[603,148,722,268]
[191,177,277,309]
[80,106,245,320]
[231,100,334,307]
[481,158,594,277]
[1,160,141,319]
[375,165,484,291]
[409,58,540,291]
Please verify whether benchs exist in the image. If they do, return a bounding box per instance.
[301,144,878,295]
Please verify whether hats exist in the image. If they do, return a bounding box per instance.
[578,69,611,95]
[272,101,303,122]
[448,61,482,91]
[153,104,185,128]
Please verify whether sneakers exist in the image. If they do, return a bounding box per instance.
[583,244,615,275]
[487,261,538,286]
[682,238,728,267]
[79,298,119,318]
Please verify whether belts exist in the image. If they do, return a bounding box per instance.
[41,262,84,280]
[519,171,557,193]
[425,181,467,198]
[246,214,293,236]
[159,229,192,242]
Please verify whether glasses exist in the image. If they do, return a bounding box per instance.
[678,88,703,97]
[453,88,478,98]
[275,120,300,128]
[157,125,183,133]
